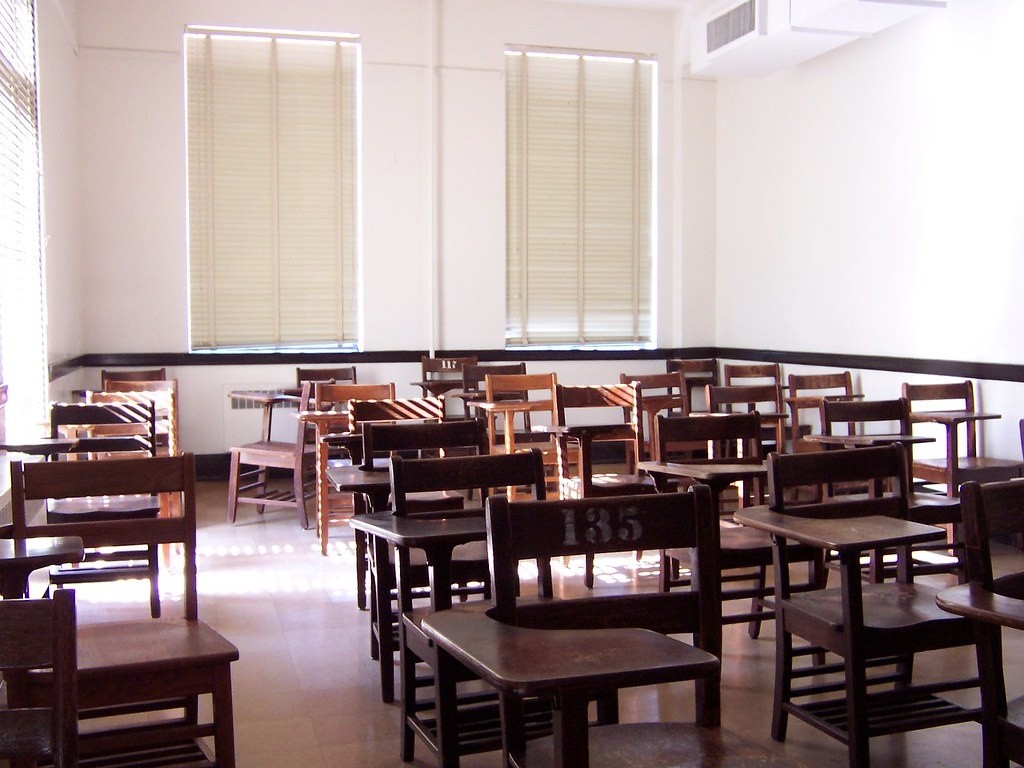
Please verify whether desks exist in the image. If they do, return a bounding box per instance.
[322,465,390,492]
[420,582,720,695]
[633,463,767,481]
[909,410,1002,423]
[801,435,937,447]
[318,433,363,446]
[0,436,80,456]
[289,410,348,423]
[228,390,315,405]
[466,401,536,412]
[349,512,485,546]
[733,498,947,550]
[547,425,613,437]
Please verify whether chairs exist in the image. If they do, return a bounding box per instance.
[0,368,239,768]
[227,356,1024,768]
